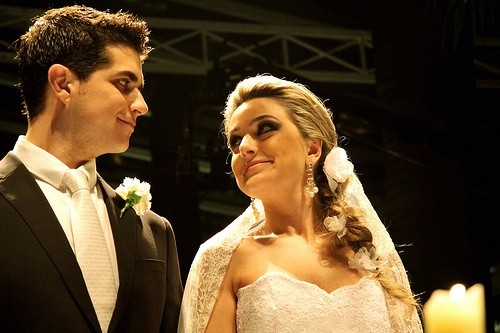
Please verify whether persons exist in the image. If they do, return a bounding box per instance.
[177,74,424,333]
[0,5,184,333]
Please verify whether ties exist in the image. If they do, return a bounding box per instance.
[61,168,118,333]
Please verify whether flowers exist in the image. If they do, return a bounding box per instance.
[348,246,384,279]
[324,216,347,240]
[114,177,152,218]
